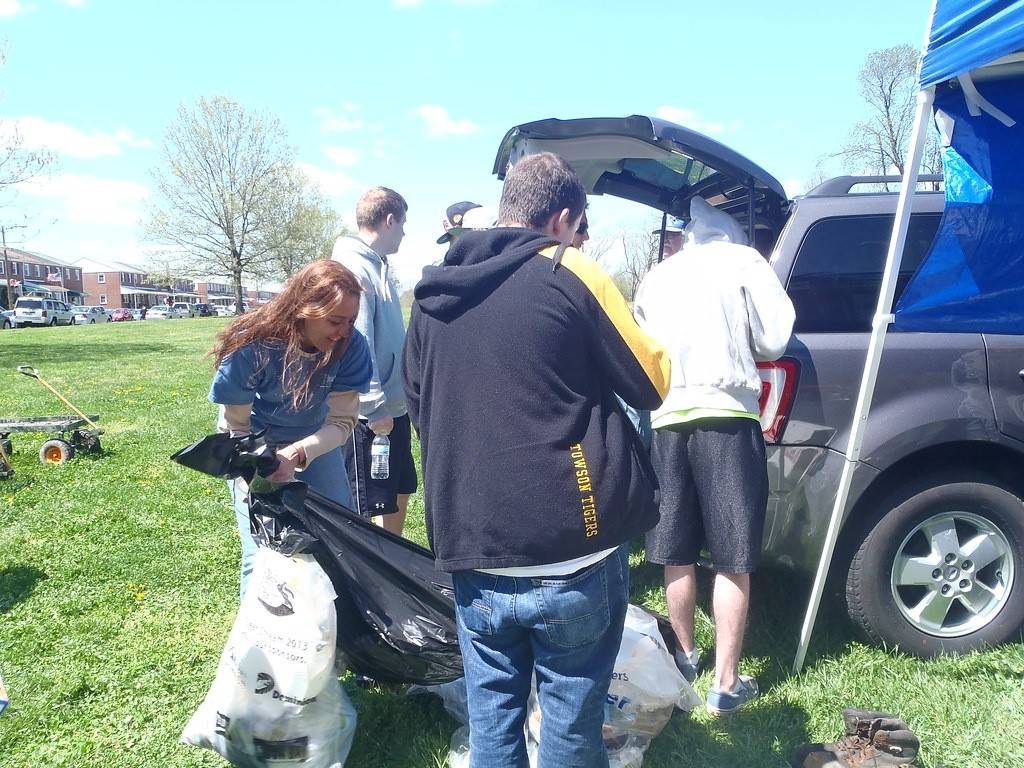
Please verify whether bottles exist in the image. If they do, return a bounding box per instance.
[369,434,389,479]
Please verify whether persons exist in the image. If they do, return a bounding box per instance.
[328,188,418,540]
[401,152,672,768]
[571,202,593,251]
[140,305,146,320]
[431,201,491,268]
[651,211,688,259]
[632,195,796,718]
[209,257,373,607]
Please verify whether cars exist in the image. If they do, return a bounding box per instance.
[145,306,182,320]
[69,306,111,324]
[231,302,256,315]
[194,303,218,316]
[173,302,197,318]
[213,306,233,316]
[111,308,133,322]
[0,312,11,329]
[2,310,15,327]
[131,309,148,320]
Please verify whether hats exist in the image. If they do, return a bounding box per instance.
[447,207,497,236]
[651,214,689,233]
[435,201,482,244]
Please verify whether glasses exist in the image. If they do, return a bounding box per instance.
[575,223,589,234]
[664,232,682,238]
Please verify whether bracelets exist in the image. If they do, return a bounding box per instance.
[289,444,301,464]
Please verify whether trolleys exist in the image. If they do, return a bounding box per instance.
[0,366,105,465]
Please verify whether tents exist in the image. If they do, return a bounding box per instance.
[778,4,1023,681]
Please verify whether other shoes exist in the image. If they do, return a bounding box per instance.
[792,709,895,767]
[678,663,699,682]
[706,674,759,713]
[802,716,920,768]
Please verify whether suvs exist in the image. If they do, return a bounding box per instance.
[13,297,75,328]
[496,115,1023,653]
